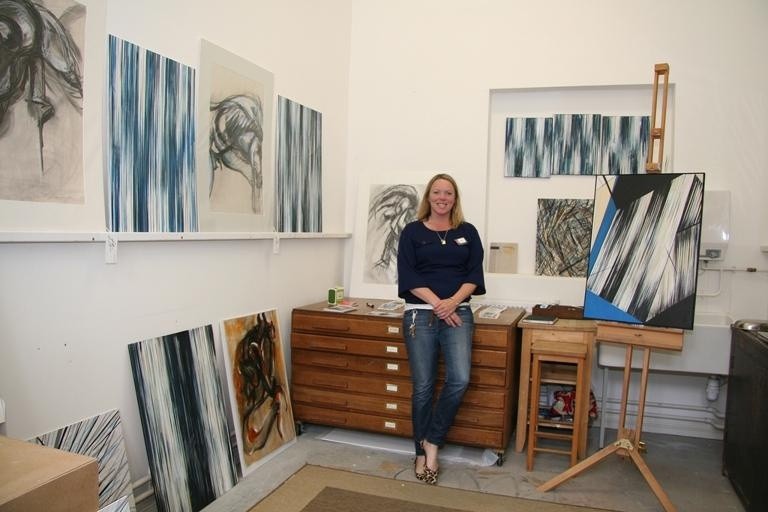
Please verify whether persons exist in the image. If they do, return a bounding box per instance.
[396,173,486,487]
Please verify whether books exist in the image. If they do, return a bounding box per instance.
[522,315,559,325]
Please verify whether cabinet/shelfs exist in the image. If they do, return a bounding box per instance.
[290,296,525,465]
[722,328,768,512]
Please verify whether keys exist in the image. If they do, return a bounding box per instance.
[409,310,418,337]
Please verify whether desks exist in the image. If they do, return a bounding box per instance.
[515,312,598,459]
[0,434,99,512]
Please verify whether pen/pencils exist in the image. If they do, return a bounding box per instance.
[366,303,374,308]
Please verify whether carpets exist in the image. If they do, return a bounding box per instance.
[246,462,621,512]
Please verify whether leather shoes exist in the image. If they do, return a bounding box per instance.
[414,440,439,484]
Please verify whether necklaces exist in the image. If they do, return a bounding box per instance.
[436,229,449,245]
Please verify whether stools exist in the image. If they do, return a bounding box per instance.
[527,340,588,478]
